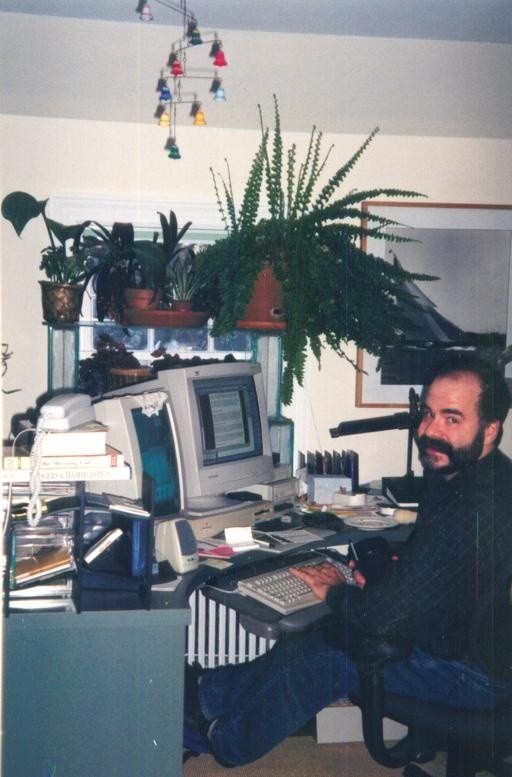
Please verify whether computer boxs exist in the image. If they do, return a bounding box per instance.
[177,500,274,540]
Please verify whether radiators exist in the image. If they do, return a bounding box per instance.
[184,588,276,669]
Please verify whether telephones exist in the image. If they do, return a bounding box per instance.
[37,393,95,432]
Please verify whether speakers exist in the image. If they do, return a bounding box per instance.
[154,517,200,575]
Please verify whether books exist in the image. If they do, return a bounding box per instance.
[3,414,132,484]
[377,472,420,510]
[15,548,78,589]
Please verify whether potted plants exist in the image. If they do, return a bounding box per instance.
[164,245,213,311]
[189,95,442,405]
[1,191,103,323]
[79,210,196,311]
[75,333,176,392]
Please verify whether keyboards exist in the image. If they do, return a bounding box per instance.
[237,556,358,616]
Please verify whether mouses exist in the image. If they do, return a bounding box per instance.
[303,511,345,530]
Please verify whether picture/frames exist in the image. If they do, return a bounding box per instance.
[355,199,511,409]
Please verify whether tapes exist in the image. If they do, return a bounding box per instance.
[333,493,365,506]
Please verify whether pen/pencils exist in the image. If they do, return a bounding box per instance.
[252,539,275,548]
[347,536,359,562]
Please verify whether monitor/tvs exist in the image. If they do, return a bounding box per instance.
[91,362,275,517]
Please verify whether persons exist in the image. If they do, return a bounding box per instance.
[183,358,512,769]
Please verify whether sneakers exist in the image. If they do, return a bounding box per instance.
[183,656,239,769]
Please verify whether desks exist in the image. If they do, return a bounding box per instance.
[2,486,419,777]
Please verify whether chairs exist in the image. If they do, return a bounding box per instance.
[347,552,511,777]
